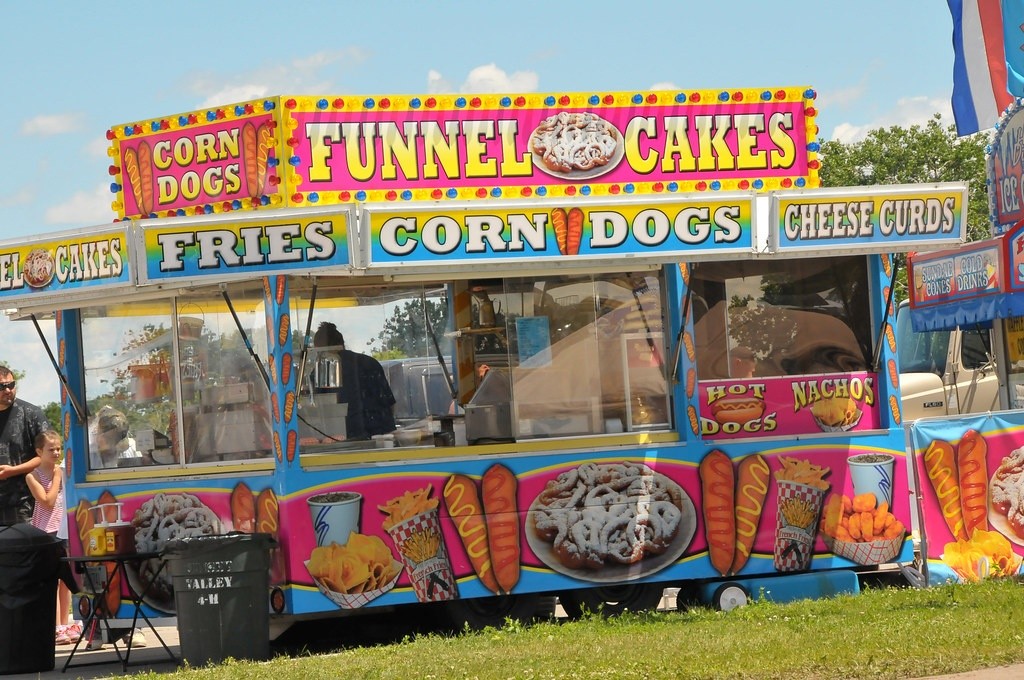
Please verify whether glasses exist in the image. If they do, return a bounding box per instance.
[0,381,15,391]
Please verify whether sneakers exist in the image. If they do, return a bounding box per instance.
[88,639,102,650]
[66,624,82,643]
[55,628,71,645]
[124,628,147,647]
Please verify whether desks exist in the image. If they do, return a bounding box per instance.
[60,550,179,673]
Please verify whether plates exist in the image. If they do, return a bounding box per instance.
[524,471,698,584]
[23,256,54,287]
[987,465,1024,547]
[527,115,625,181]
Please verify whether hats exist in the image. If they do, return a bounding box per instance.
[99,408,130,451]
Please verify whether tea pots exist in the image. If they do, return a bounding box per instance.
[468,293,501,328]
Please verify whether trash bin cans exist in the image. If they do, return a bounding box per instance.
[161,527,276,668]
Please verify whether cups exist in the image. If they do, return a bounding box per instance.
[387,508,459,602]
[306,491,364,549]
[847,453,895,513]
[774,480,825,573]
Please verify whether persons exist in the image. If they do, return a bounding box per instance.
[26,404,147,651]
[0,365,50,530]
[310,321,397,442]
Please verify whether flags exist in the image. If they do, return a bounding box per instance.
[946,0,1024,138]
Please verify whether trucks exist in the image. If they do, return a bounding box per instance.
[0,85,1023,657]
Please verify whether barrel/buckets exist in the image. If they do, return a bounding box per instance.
[179,303,204,342]
